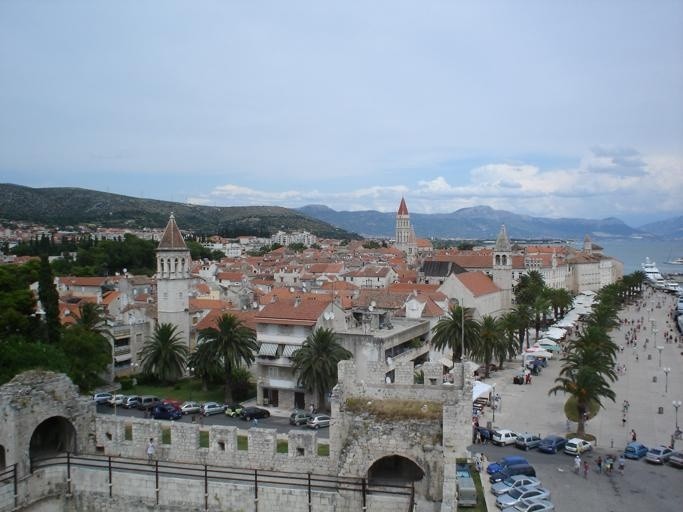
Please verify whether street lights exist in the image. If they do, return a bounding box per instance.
[672,401,681,427]
[657,346,664,367]
[663,368,670,392]
[651,330,658,347]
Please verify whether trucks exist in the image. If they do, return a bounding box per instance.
[456,463,476,507]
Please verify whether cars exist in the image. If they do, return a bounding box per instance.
[487,456,554,512]
[624,442,683,466]
[290,413,330,429]
[93,393,270,421]
[492,430,592,456]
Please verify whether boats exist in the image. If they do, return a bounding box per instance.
[663,258,683,265]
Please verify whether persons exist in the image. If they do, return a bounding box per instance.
[605,457,609,476]
[479,453,484,470]
[623,400,626,412]
[597,456,602,473]
[308,403,313,414]
[622,413,626,427]
[609,456,613,473]
[630,429,636,442]
[574,455,580,474]
[475,429,482,446]
[670,435,675,448]
[566,417,570,431]
[252,416,257,427]
[583,461,588,479]
[144,438,156,464]
[482,428,490,445]
[246,416,250,425]
[618,456,624,476]
